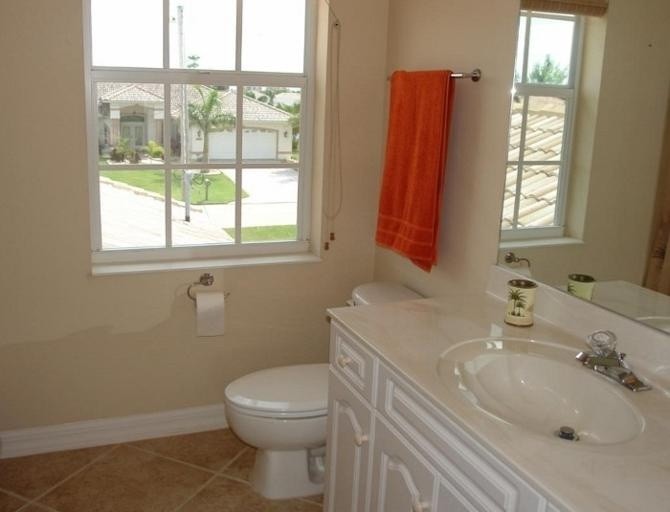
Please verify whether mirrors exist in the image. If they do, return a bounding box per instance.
[498,0,669,340]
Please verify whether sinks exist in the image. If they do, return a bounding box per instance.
[638,317,670,336]
[457,353,645,443]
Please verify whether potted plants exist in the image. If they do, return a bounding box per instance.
[567,273,595,302]
[504,277,538,326]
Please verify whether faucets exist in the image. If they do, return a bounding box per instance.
[576,329,652,392]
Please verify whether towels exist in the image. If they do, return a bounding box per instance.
[376,67,451,273]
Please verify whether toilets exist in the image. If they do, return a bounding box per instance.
[225,282,430,501]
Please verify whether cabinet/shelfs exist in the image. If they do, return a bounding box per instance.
[322,318,566,512]
